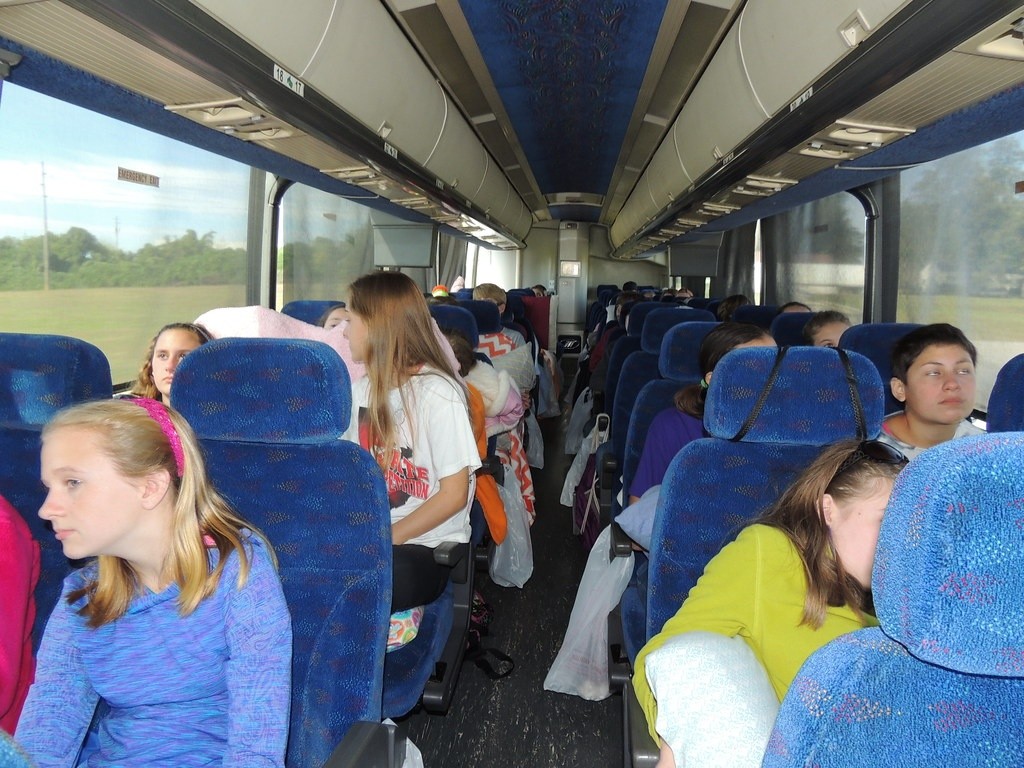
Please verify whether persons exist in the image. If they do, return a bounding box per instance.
[0,271,547,768]
[588,281,987,768]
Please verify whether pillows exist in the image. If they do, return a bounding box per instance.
[643,631,781,767]
[490,342,537,391]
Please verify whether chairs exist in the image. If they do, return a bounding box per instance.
[0,284,1024,768]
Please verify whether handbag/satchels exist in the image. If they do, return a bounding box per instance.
[564,386,594,455]
[524,397,545,470]
[542,521,635,701]
[468,589,490,637]
[559,413,610,507]
[537,356,562,419]
[487,464,534,588]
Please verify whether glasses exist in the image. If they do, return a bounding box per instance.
[833,440,911,477]
